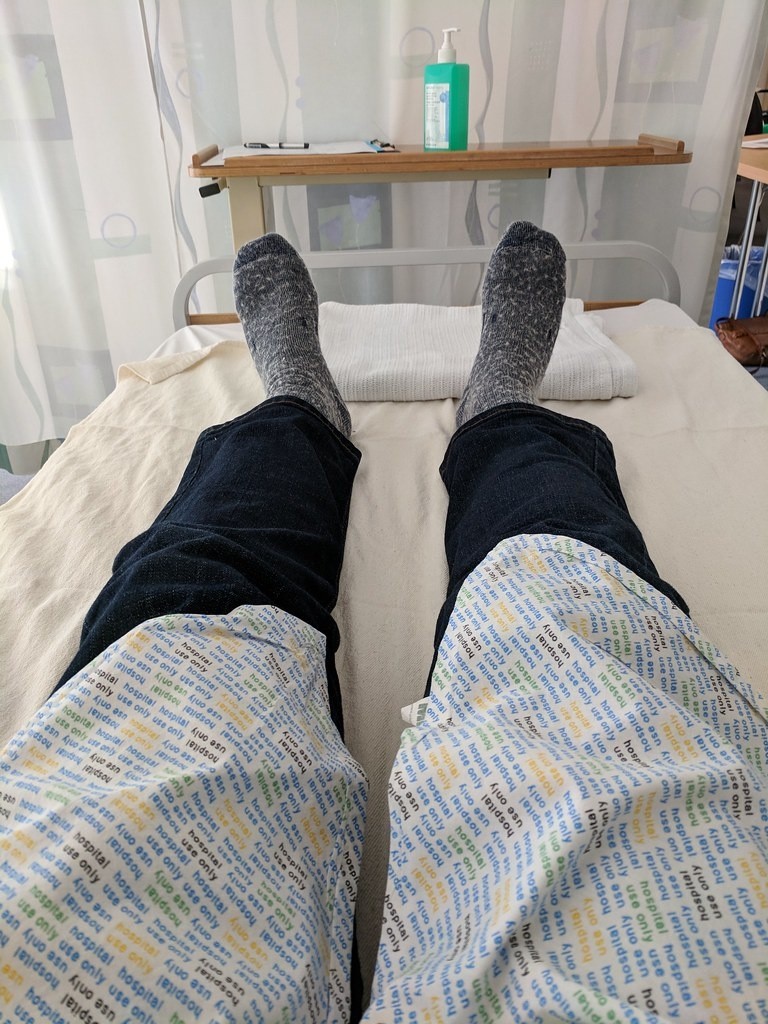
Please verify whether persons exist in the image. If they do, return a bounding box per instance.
[0,221,767,1024]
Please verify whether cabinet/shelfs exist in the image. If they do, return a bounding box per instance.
[186,130,693,255]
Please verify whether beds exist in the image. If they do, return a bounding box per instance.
[2,237,768,1024]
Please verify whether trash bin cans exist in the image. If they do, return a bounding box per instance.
[709,243,767,336]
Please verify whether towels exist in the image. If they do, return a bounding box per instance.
[292,300,639,400]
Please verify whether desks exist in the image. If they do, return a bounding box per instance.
[729,132,768,320]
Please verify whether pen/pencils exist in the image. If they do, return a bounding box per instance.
[243,142,309,150]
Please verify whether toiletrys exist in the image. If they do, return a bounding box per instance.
[422,26,470,153]
